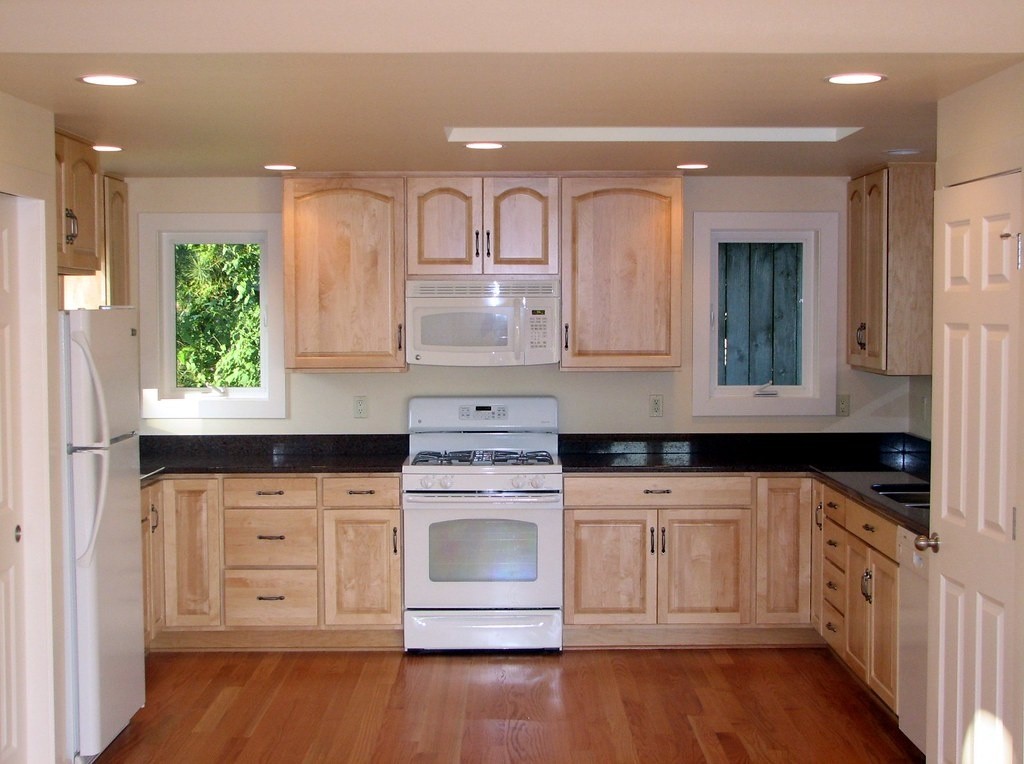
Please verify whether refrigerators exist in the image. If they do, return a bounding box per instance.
[52,307,146,764]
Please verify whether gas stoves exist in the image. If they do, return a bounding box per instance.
[402,397,563,493]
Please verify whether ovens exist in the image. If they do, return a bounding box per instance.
[403,490,563,652]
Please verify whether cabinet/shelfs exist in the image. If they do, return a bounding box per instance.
[153,474,225,628]
[54,131,105,277]
[752,476,816,627]
[567,475,758,628]
[135,491,168,643]
[284,185,402,373]
[407,178,561,273]
[68,176,135,307]
[324,475,404,631]
[847,164,932,376]
[226,475,324,629]
[562,171,684,369]
[814,496,904,712]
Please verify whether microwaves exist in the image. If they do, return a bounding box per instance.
[403,279,561,367]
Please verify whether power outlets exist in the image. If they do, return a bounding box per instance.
[920,395,927,421]
[836,394,850,418]
[352,395,369,420]
[647,394,663,416]
